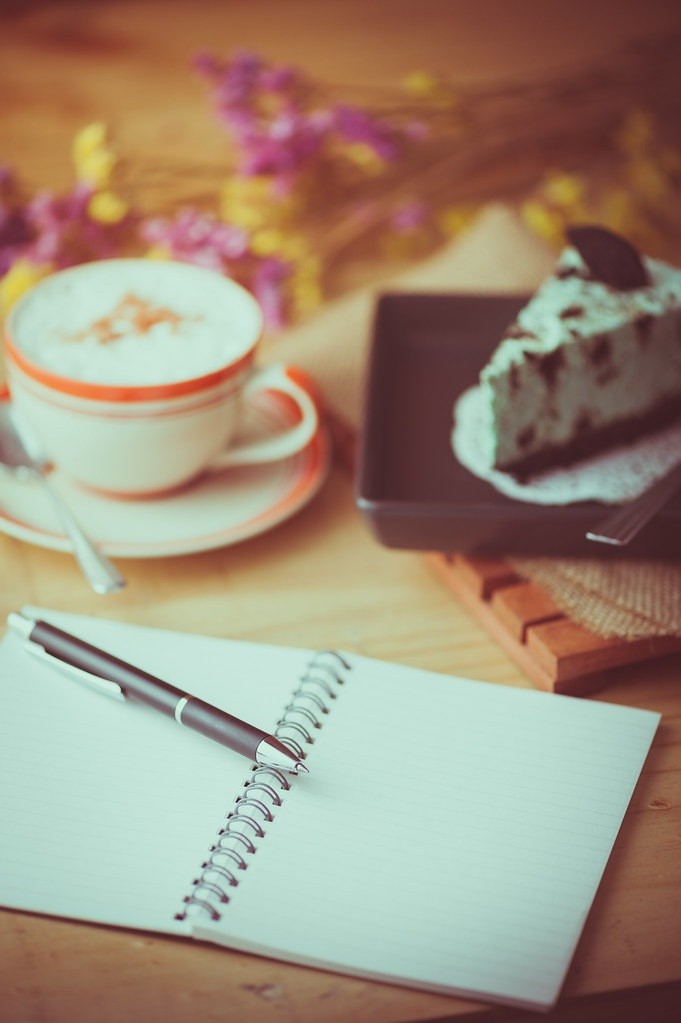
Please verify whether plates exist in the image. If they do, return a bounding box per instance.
[0,360,332,560]
[353,281,681,560]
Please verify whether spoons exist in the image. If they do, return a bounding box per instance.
[0,413,129,594]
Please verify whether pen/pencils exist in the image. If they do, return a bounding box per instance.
[5,609,312,776]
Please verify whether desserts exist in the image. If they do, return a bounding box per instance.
[475,219,681,480]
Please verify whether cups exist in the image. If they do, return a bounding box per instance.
[1,255,319,503]
